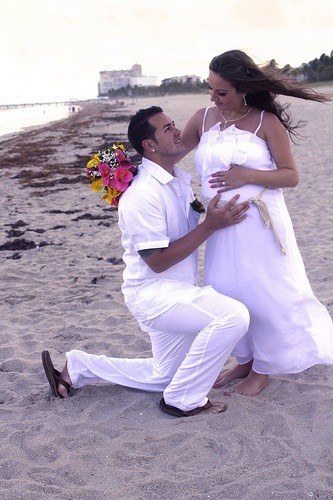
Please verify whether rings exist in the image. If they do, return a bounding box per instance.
[222,181,226,186]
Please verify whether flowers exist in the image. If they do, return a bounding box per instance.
[189,199,205,213]
[87,143,140,208]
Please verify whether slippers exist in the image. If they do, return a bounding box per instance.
[42,350,72,399]
[159,396,227,417]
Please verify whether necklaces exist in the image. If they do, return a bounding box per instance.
[220,107,253,129]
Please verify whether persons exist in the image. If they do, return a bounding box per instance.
[172,48,332,399]
[42,106,251,417]
